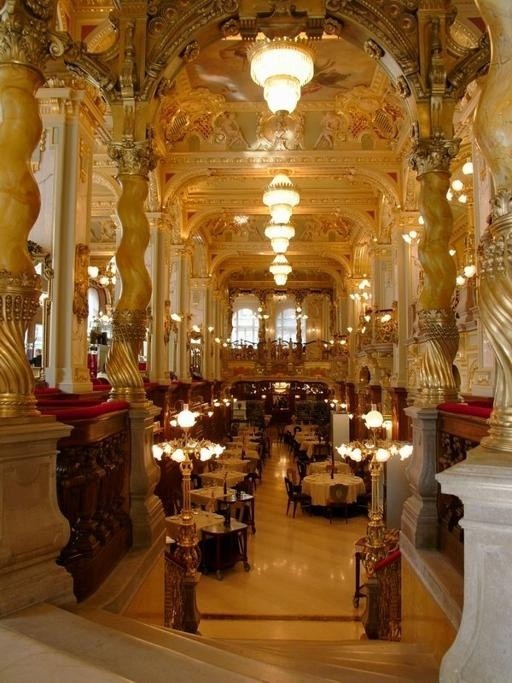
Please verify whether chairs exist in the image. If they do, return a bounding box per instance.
[161,414,364,567]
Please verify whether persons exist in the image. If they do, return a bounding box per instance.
[29,348,42,368]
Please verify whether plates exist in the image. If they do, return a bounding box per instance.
[305,468,361,485]
[170,511,223,520]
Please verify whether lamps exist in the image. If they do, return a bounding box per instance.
[334,403,415,639]
[152,404,227,634]
[235,34,327,291]
[381,301,398,348]
[73,243,118,323]
[164,299,184,345]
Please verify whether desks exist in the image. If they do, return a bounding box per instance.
[198,519,251,581]
